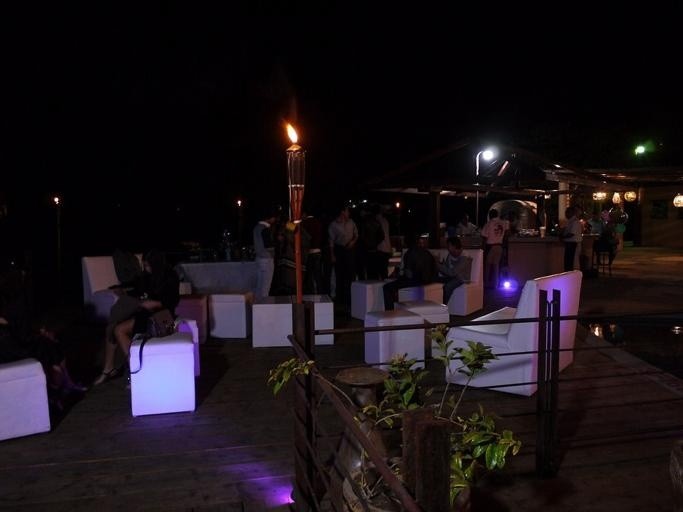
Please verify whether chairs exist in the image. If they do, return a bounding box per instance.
[443,268,582,397]
[81,254,124,321]
[400,248,484,316]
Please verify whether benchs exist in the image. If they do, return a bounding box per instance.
[252,294,334,347]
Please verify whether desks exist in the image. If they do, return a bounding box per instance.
[176,260,258,299]
[591,240,616,276]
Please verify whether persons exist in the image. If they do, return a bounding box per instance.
[254,199,624,310]
[92,252,179,386]
[0,303,91,417]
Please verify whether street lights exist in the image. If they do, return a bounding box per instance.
[475,147,495,226]
[283,101,317,358]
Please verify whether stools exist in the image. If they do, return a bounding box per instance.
[131,329,196,417]
[175,293,206,342]
[206,291,254,338]
[0,359,51,443]
[363,311,424,373]
[351,279,393,320]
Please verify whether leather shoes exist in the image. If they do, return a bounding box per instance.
[64,383,89,394]
[93,367,119,387]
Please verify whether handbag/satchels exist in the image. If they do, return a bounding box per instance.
[124,308,175,374]
